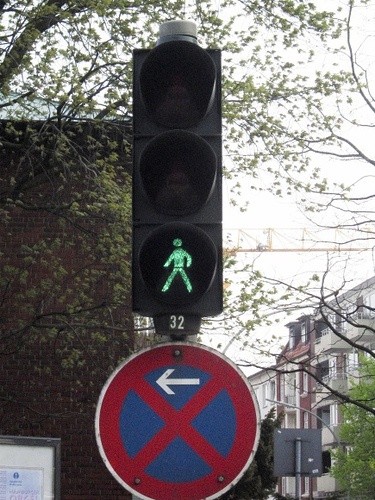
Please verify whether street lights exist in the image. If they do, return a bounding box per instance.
[266,397,344,454]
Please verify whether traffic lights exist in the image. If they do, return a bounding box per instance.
[131,40,224,314]
